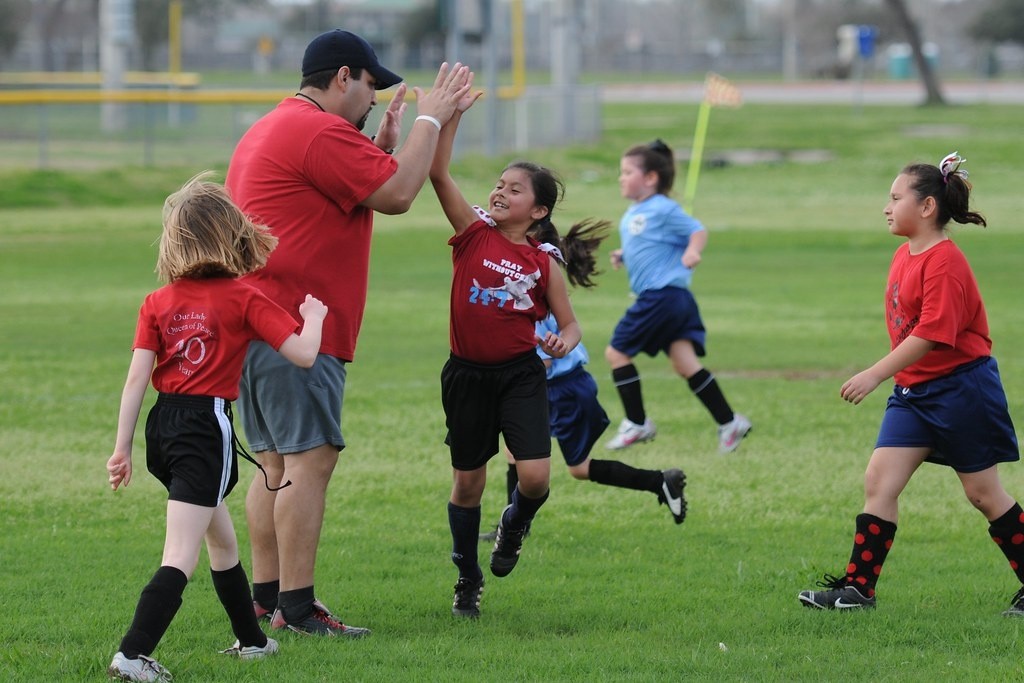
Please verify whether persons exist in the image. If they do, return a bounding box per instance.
[479,220,687,542]
[605,139,752,452]
[106,171,328,683]
[429,65,583,620]
[799,165,1024,617]
[223,30,472,640]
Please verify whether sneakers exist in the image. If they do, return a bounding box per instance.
[1001,586,1024,618]
[109,651,173,682]
[662,468,688,525]
[489,503,527,578]
[452,574,485,615]
[233,637,279,660]
[718,413,752,454]
[252,598,373,641]
[606,418,656,449]
[798,573,876,613]
[479,525,531,543]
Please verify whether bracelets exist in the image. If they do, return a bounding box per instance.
[414,115,443,131]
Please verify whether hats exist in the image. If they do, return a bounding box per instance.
[302,28,403,90]
